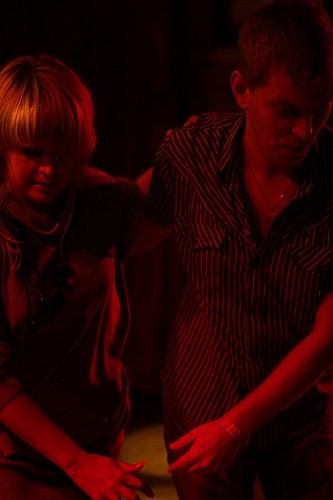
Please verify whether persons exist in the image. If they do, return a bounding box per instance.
[0,55,200,500]
[80,0,333,500]
